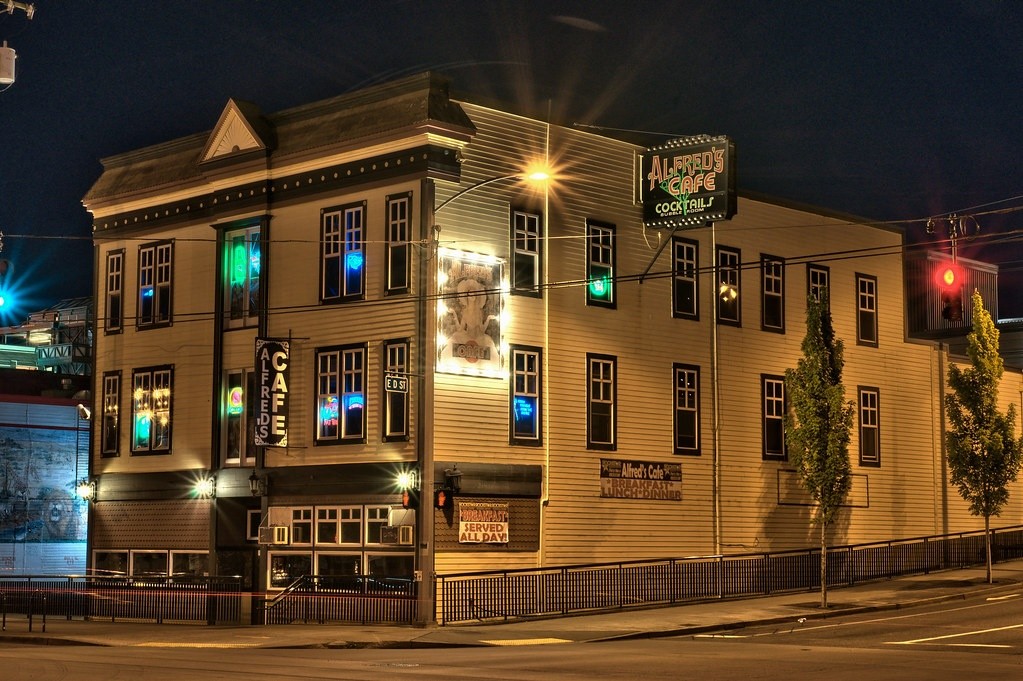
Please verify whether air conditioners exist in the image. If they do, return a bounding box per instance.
[380,525,413,546]
[258,526,289,545]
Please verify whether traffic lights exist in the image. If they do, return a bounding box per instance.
[433,488,450,510]
[401,489,419,510]
[935,264,959,289]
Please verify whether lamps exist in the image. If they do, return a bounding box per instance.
[443,465,464,493]
[78,477,97,502]
[247,469,268,497]
